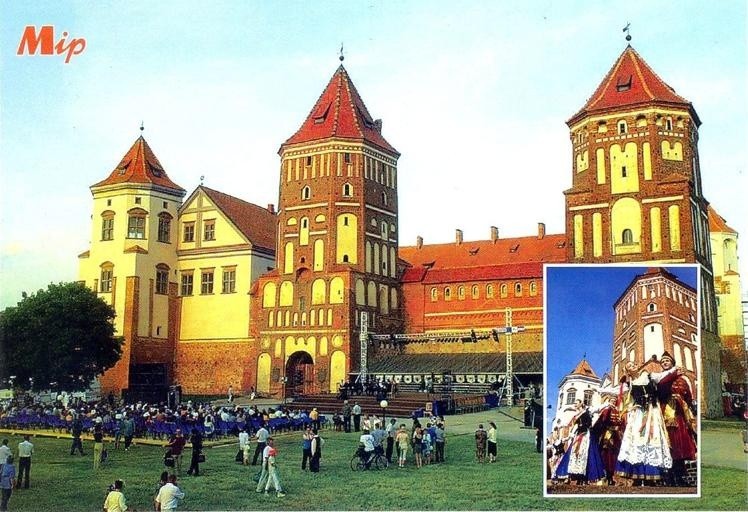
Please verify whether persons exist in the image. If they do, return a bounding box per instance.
[255,437,287,494]
[264,448,286,497]
[1,391,327,477]
[227,385,233,403]
[474,424,488,463]
[547,351,698,489]
[521,383,542,400]
[103,483,116,503]
[331,373,447,470]
[153,475,185,512]
[0,455,16,512]
[152,471,169,512]
[15,435,33,490]
[249,386,256,401]
[252,384,255,391]
[102,479,129,512]
[0,437,11,472]
[486,422,497,464]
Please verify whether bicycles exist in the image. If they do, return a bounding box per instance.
[348,443,389,473]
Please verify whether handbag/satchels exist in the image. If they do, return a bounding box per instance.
[236,450,243,462]
[199,455,205,463]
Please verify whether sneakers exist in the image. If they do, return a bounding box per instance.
[489,460,493,463]
[125,449,127,451]
[265,490,269,494]
[492,459,496,462]
[187,471,191,474]
[194,474,199,477]
[387,460,445,468]
[256,489,262,493]
[551,479,692,487]
[127,447,130,449]
[276,493,285,498]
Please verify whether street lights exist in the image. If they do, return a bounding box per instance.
[279,376,289,405]
